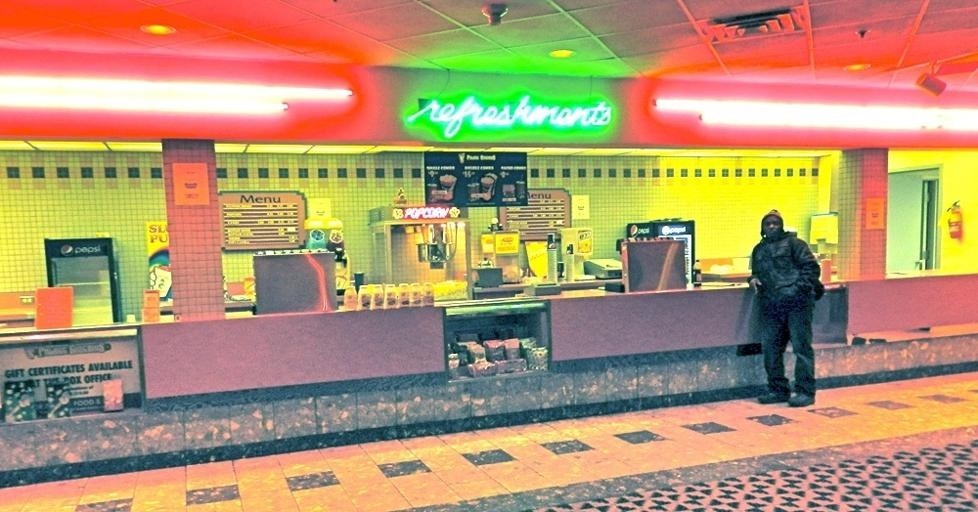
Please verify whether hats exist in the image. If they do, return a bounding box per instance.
[762,208,783,223]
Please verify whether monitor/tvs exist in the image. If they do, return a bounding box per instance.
[620,239,686,293]
[253,252,338,313]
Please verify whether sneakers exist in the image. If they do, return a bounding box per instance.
[787,393,815,407]
[755,391,791,404]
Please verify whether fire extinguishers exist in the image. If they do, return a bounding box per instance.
[946,200,963,238]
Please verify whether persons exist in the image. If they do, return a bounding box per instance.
[746,208,819,407]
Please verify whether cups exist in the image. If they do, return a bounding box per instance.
[547,233,558,283]
[564,242,574,283]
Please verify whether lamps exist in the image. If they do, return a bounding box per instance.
[482,4,508,26]
[917,60,947,95]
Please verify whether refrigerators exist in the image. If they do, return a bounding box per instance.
[623,219,695,285]
[42,236,121,325]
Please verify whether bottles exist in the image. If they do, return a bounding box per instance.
[692,259,702,287]
[343,282,435,311]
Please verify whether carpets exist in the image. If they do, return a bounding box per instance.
[523,441,978,512]
